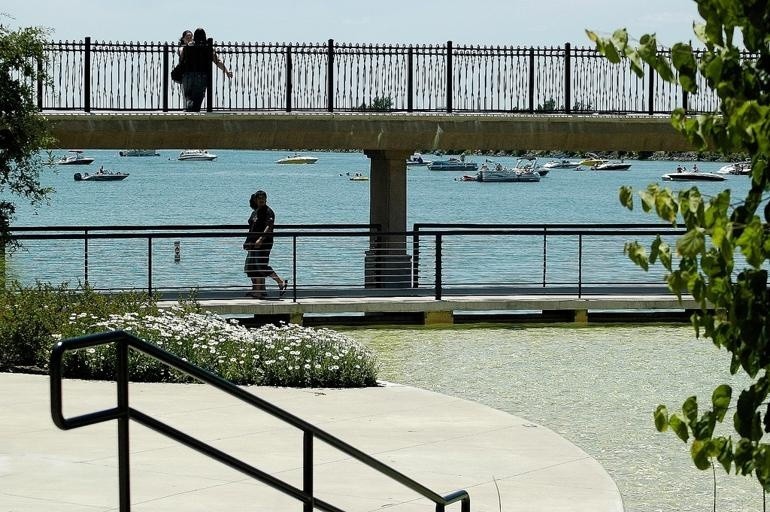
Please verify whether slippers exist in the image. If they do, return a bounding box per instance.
[280,280,288,296]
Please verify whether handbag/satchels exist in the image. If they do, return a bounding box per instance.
[170,64,183,84]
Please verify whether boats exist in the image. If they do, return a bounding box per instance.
[74,165,130,181]
[56,150,95,165]
[274,156,319,164]
[660,158,751,180]
[119,149,161,157]
[406,152,632,182]
[350,176,369,181]
[177,149,218,161]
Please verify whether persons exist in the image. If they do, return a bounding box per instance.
[242,194,268,297]
[249,190,289,299]
[412,150,625,183]
[99,165,105,176]
[682,166,686,173]
[692,163,698,172]
[61,154,67,163]
[676,164,683,173]
[178,28,233,112]
[287,153,301,158]
[736,164,744,174]
[177,30,196,112]
[339,171,362,177]
